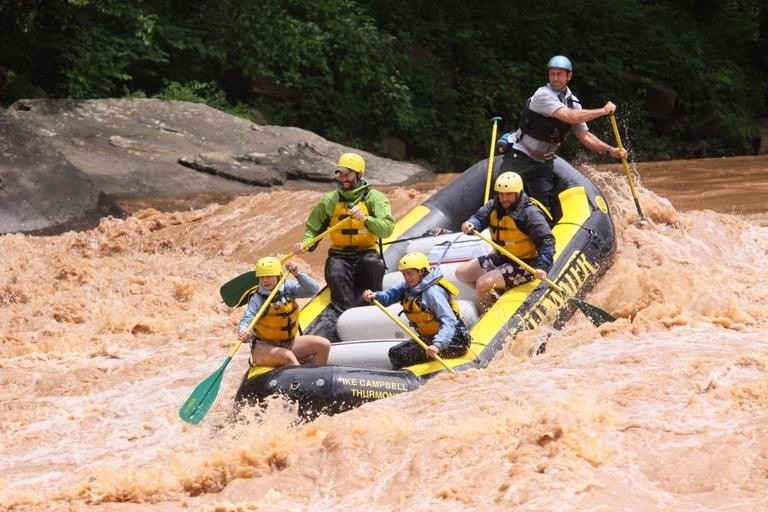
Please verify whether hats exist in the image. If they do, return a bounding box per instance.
[334,166,353,175]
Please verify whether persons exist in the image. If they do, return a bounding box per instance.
[362,252,472,371]
[292,152,395,317]
[454,171,556,316]
[496,55,628,230]
[237,256,331,367]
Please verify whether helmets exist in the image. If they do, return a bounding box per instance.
[548,55,573,72]
[255,256,283,277]
[398,251,430,282]
[336,152,366,178]
[494,170,525,198]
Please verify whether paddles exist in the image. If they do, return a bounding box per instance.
[178,270,290,426]
[471,229,617,328]
[220,215,354,309]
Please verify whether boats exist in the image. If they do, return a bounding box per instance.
[234,150,617,412]
